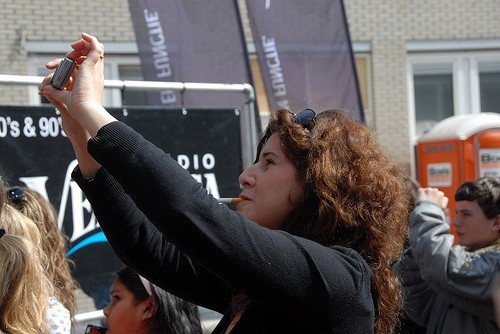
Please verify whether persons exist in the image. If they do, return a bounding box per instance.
[104,266,201,332]
[37,32,413,332]
[0,175,78,332]
[394,174,500,334]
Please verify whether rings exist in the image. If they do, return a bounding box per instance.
[99,55,104,60]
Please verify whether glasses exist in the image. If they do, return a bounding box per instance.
[293,109,316,126]
[7,186,26,211]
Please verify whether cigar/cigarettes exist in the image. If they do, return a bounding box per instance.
[217,198,244,205]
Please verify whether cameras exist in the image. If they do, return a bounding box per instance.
[50,58,76,90]
